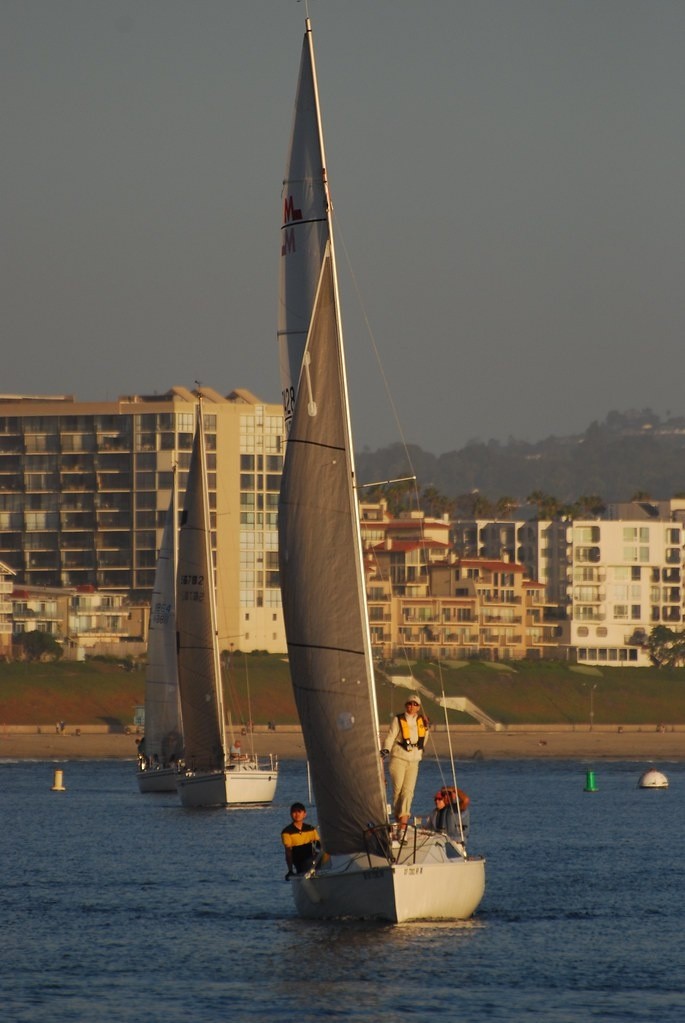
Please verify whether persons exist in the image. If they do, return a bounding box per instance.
[135,737,145,756]
[55,719,64,735]
[281,802,332,882]
[247,717,254,728]
[125,726,130,731]
[425,786,468,846]
[380,694,429,844]
[269,717,274,729]
[230,740,241,756]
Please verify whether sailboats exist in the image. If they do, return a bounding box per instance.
[276,16,491,922]
[135,459,196,795]
[175,394,278,809]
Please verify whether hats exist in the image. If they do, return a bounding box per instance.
[435,791,442,799]
[291,803,305,811]
[405,696,421,705]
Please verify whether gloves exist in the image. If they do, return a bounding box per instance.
[380,749,389,759]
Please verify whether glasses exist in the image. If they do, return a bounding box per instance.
[408,702,418,706]
[441,791,452,797]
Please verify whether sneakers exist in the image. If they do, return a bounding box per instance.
[393,834,398,840]
[396,828,408,845]
